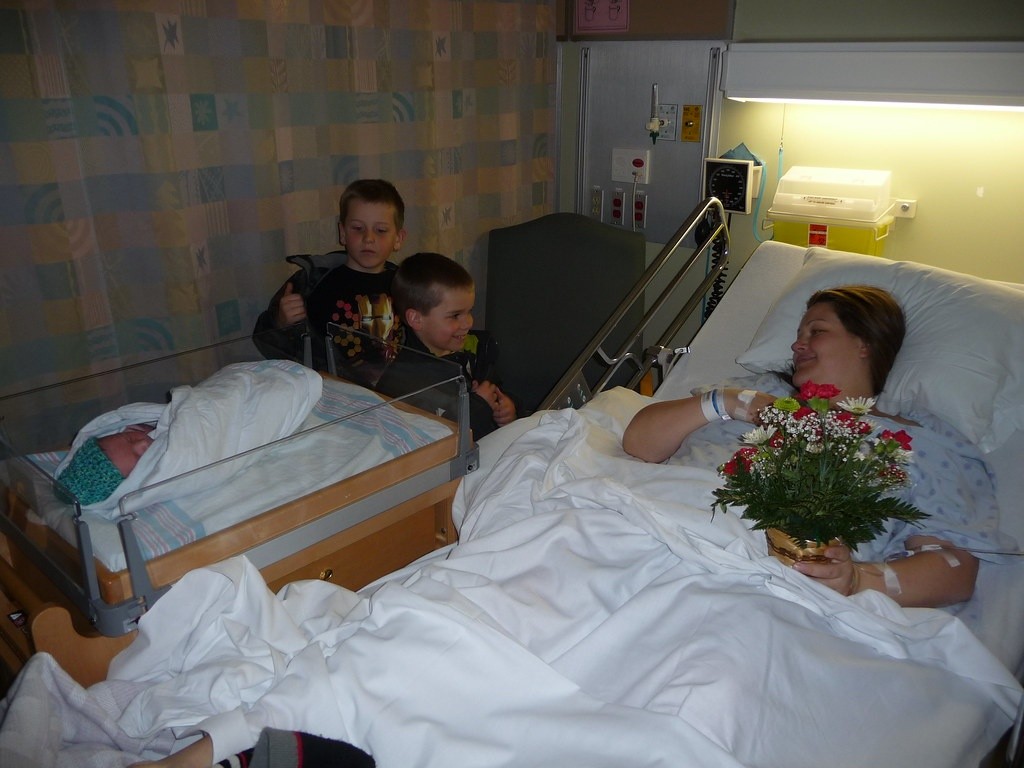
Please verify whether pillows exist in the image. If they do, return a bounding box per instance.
[736,247,1024,459]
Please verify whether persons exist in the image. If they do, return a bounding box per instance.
[252,178,408,390]
[106,285,1024,767]
[52,360,322,506]
[374,252,523,443]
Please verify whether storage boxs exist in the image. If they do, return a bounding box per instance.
[760,215,894,256]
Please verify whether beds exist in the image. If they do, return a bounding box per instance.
[5,363,475,602]
[0,240,1023,768]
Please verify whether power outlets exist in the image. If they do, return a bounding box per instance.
[611,192,625,225]
[610,148,647,185]
[632,194,649,229]
[591,190,604,222]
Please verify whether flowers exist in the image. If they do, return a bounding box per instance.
[708,379,932,552]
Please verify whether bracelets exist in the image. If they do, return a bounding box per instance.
[700,388,733,422]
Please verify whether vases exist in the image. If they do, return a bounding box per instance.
[762,525,841,569]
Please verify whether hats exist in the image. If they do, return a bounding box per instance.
[53,437,125,506]
